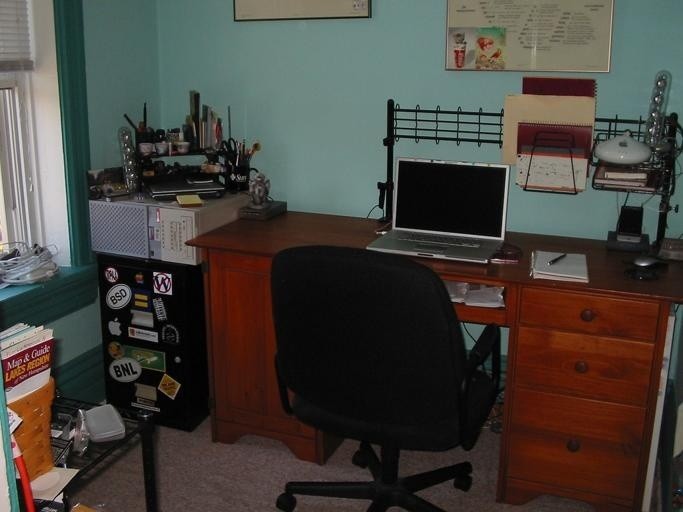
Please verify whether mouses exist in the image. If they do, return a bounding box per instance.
[633,256,668,272]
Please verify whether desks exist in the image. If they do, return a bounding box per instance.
[185,205,683,512]
[29,396,159,512]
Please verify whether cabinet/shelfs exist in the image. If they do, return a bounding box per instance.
[95,251,211,433]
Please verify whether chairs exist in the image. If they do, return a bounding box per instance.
[269,246,501,510]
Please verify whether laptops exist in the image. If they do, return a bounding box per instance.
[365,157,510,265]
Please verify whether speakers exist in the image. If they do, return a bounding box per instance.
[617,205,643,243]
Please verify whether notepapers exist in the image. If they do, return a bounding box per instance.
[176,195,203,205]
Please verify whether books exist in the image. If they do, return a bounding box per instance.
[501,76,662,192]
[531,250,588,283]
[144,173,226,200]
[0,321,54,406]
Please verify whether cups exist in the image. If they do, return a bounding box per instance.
[139,142,153,156]
[176,141,190,153]
[155,142,168,155]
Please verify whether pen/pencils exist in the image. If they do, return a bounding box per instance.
[547,253,566,266]
[236,138,261,167]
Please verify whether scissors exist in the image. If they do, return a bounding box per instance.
[220,138,237,167]
